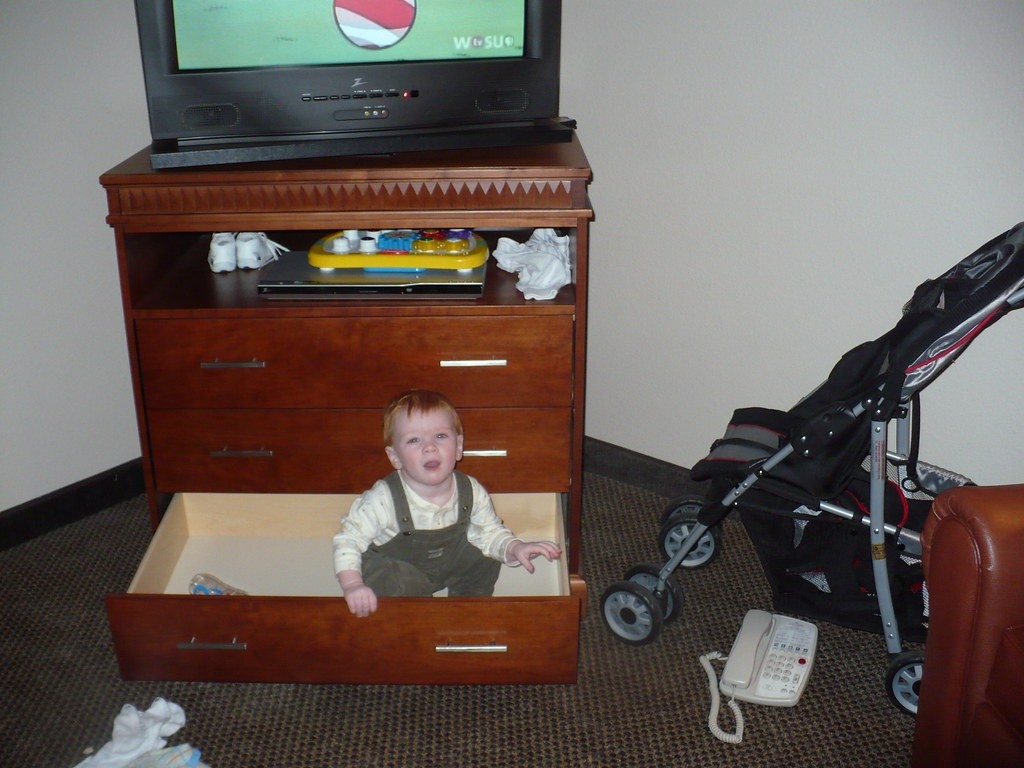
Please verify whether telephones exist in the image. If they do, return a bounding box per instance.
[719,609,818,707]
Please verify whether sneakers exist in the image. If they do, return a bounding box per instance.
[208,232,290,273]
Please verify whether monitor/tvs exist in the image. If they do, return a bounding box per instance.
[132,0,577,171]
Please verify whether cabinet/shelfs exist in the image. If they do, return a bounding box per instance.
[99,116,596,686]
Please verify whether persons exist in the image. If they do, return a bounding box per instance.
[331,389,563,617]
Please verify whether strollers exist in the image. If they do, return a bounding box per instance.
[600,222,1024,722]
